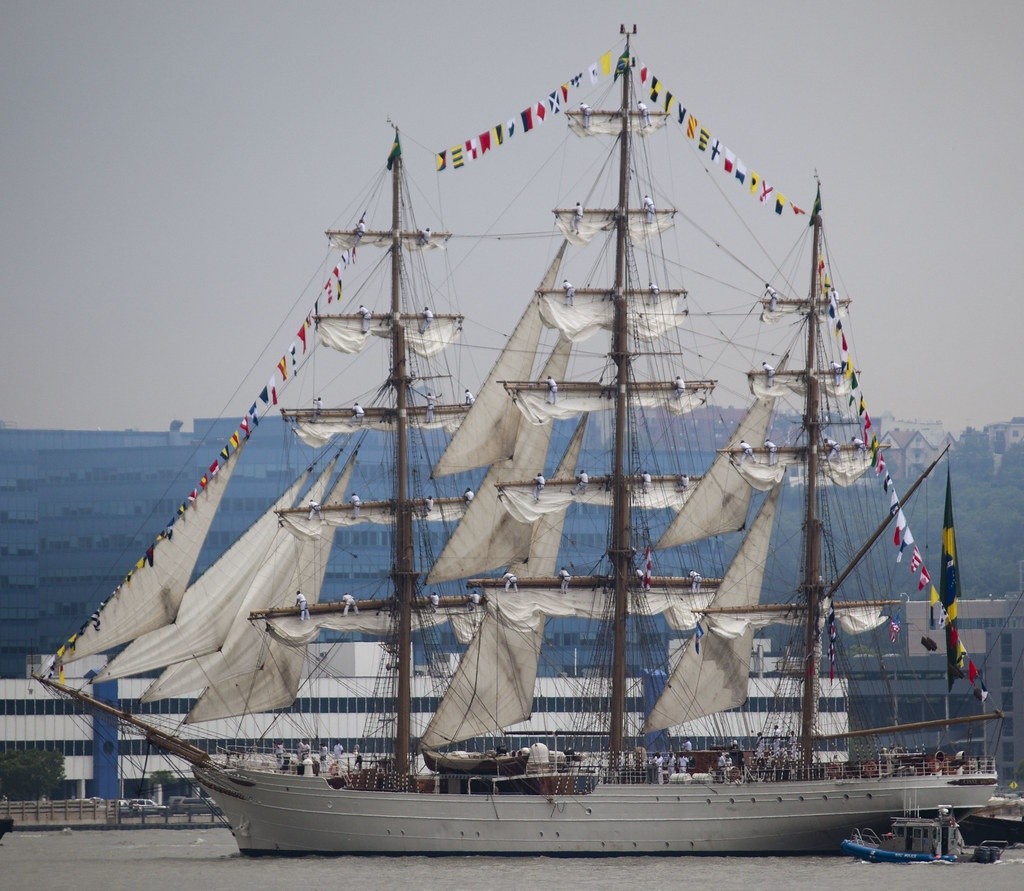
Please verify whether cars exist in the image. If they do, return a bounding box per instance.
[119,800,141,815]
[73,797,106,807]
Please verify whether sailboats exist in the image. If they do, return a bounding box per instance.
[29,23,1011,857]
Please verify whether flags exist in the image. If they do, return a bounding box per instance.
[937,459,963,695]
[38,51,988,703]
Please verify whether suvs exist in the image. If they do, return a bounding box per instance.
[168,796,185,812]
[131,799,169,816]
[180,797,223,815]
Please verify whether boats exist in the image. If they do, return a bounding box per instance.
[422,749,531,775]
[839,816,1008,863]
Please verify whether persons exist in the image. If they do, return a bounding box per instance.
[763,439,777,466]
[570,470,589,496]
[307,499,323,520]
[340,593,358,617]
[688,569,702,596]
[851,437,867,460]
[533,473,545,503]
[676,474,689,494]
[349,492,360,519]
[425,591,439,613]
[501,571,519,593]
[295,590,311,620]
[464,590,479,611]
[275,724,797,785]
[735,441,756,466]
[558,567,570,595]
[634,568,645,593]
[421,496,433,517]
[824,439,841,463]
[465,487,474,506]
[641,471,651,494]
